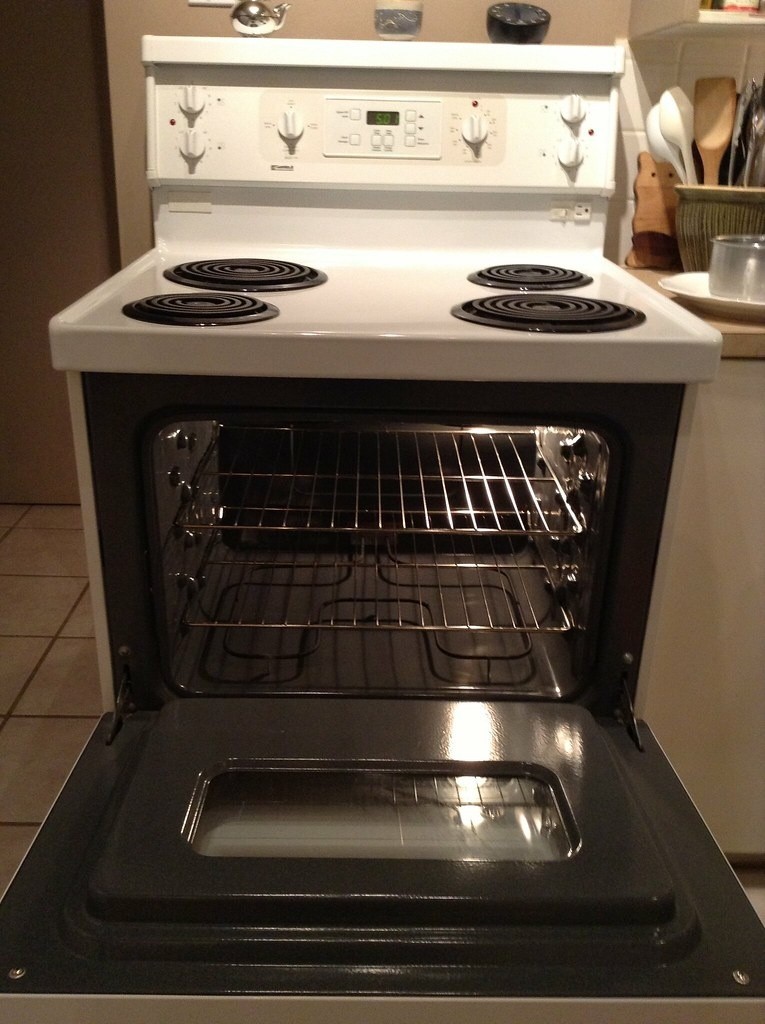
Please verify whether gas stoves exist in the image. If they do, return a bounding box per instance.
[48,35,723,384]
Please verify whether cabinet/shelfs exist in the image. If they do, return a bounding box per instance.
[627,0,765,41]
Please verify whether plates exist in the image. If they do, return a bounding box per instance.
[657,272,765,323]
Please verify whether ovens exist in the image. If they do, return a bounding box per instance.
[0,372,765,1024]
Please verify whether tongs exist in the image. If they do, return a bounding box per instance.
[727,78,758,186]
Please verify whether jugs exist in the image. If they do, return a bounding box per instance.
[231,0,292,36]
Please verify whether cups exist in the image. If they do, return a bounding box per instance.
[708,234,765,302]
[375,0,423,41]
[674,184,765,272]
